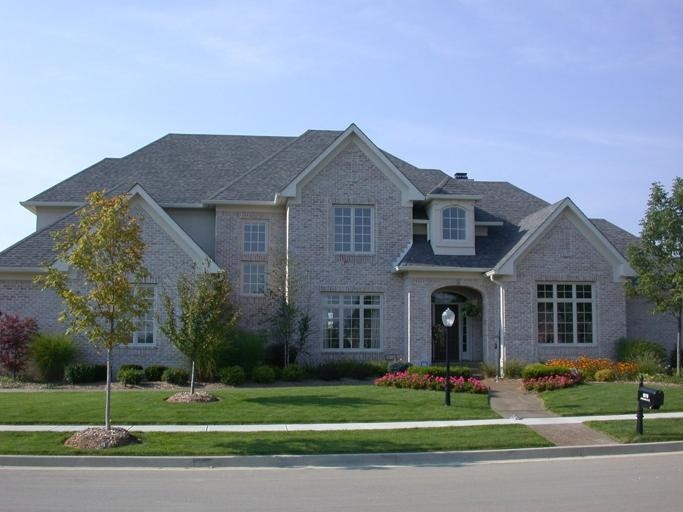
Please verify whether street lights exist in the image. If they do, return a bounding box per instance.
[440,307,455,407]
